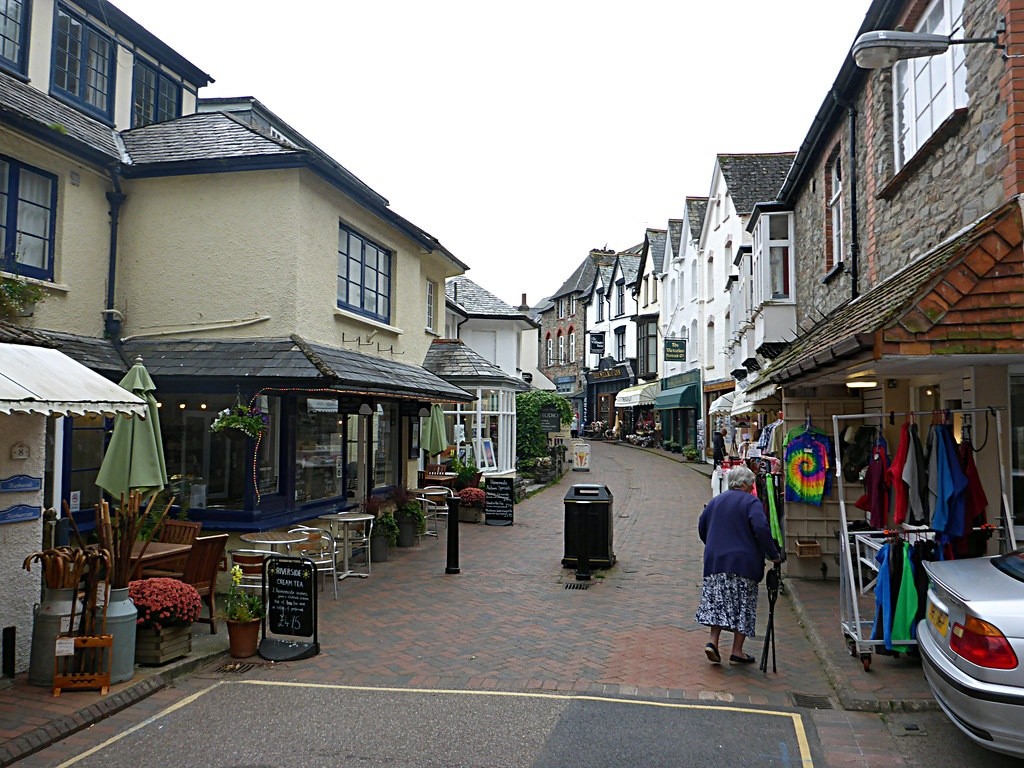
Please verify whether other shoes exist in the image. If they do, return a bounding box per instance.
[705,643,721,663]
[730,651,755,664]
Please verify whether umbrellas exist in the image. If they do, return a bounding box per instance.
[95,354,167,505]
[615,415,619,439]
[422,403,448,473]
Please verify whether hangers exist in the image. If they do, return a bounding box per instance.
[876,409,943,430]
[880,529,928,544]
[776,410,813,434]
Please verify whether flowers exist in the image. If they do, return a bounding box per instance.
[453,487,486,507]
[0,228,60,321]
[126,576,203,631]
[211,565,268,622]
[207,404,266,438]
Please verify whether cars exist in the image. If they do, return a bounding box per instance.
[914,545,1024,760]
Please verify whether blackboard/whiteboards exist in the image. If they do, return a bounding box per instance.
[485,477,513,521]
[261,556,316,641]
[539,412,561,431]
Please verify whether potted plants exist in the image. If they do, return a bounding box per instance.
[391,502,427,547]
[663,440,672,450]
[681,445,700,460]
[671,442,681,453]
[365,512,399,564]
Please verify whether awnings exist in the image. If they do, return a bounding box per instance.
[731,384,782,415]
[709,390,742,415]
[614,382,657,407]
[0,340,148,420]
[655,384,696,410]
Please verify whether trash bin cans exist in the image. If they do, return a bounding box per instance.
[562,485,616,570]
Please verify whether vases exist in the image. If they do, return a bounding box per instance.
[223,428,244,440]
[458,505,481,522]
[225,617,262,659]
[135,623,192,665]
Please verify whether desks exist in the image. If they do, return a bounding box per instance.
[316,514,376,579]
[84,540,192,583]
[241,532,309,553]
[413,490,450,538]
[425,475,455,490]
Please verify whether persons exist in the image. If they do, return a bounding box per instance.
[588,424,612,439]
[698,466,781,664]
[571,414,579,438]
[713,429,728,471]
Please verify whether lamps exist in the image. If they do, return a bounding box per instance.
[852,15,1005,68]
[359,404,372,415]
[418,408,430,416]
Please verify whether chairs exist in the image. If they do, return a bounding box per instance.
[181,534,229,635]
[424,486,454,533]
[418,464,447,488]
[285,511,367,600]
[414,498,439,540]
[143,521,202,578]
[226,548,281,589]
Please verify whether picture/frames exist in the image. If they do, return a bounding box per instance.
[457,437,498,472]
